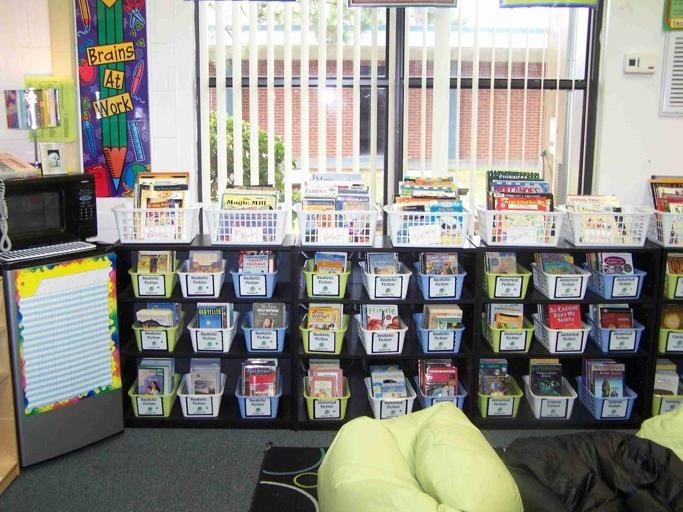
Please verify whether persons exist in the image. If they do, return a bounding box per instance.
[47,149,62,168]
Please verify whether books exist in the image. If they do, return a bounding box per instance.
[530,252,581,396]
[238,249,285,396]
[418,252,463,396]
[135,170,683,247]
[585,250,634,396]
[360,252,408,399]
[308,252,347,399]
[138,250,175,393]
[478,250,523,395]
[654,253,683,396]
[189,250,233,395]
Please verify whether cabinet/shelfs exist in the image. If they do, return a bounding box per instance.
[113,247,295,429]
[473,246,661,428]
[294,245,473,429]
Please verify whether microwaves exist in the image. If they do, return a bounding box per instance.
[0,174,100,251]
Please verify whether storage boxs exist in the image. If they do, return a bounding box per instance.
[177,268,226,297]
[659,305,682,353]
[179,373,228,417]
[242,312,290,353]
[413,313,465,354]
[664,253,683,299]
[361,268,413,300]
[480,313,536,353]
[576,376,637,418]
[235,375,283,417]
[130,311,186,353]
[475,376,523,418]
[128,374,181,417]
[414,376,468,411]
[522,375,579,418]
[228,268,280,297]
[303,376,351,419]
[354,313,409,355]
[127,268,177,298]
[301,269,353,298]
[586,312,645,353]
[416,268,467,299]
[187,309,239,354]
[363,377,417,417]
[298,313,350,355]
[531,314,591,353]
[533,268,593,301]
[588,268,647,300]
[652,359,682,417]
[481,268,533,300]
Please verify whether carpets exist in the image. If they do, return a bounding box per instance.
[246,446,326,511]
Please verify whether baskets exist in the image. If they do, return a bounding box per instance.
[111,203,682,246]
[648,207,683,247]
[112,203,203,244]
[207,203,289,245]
[476,204,564,246]
[293,203,381,246]
[559,205,653,246]
[384,203,473,247]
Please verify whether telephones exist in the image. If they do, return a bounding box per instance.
[0,151,38,178]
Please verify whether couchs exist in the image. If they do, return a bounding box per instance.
[636,403,683,461]
[489,431,683,511]
[317,400,524,510]
